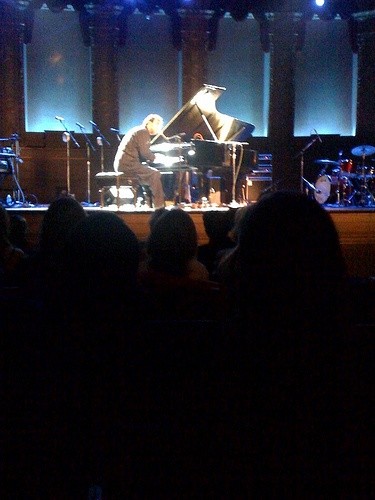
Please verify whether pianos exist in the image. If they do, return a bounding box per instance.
[142,82,257,209]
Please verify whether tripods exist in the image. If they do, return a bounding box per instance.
[347,147,375,203]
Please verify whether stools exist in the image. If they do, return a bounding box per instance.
[95,172,152,209]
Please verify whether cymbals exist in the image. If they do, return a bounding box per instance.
[312,159,338,166]
[351,145,375,157]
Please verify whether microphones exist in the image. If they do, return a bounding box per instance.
[110,128,119,132]
[315,188,321,193]
[89,121,96,126]
[55,117,64,120]
[165,133,185,140]
[314,129,322,143]
[76,122,84,128]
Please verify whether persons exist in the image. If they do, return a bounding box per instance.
[113,114,165,209]
[0,188,375,500]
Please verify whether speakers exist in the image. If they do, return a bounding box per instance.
[241,174,273,203]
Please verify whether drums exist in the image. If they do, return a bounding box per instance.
[355,165,373,187]
[313,174,348,205]
[340,158,357,174]
